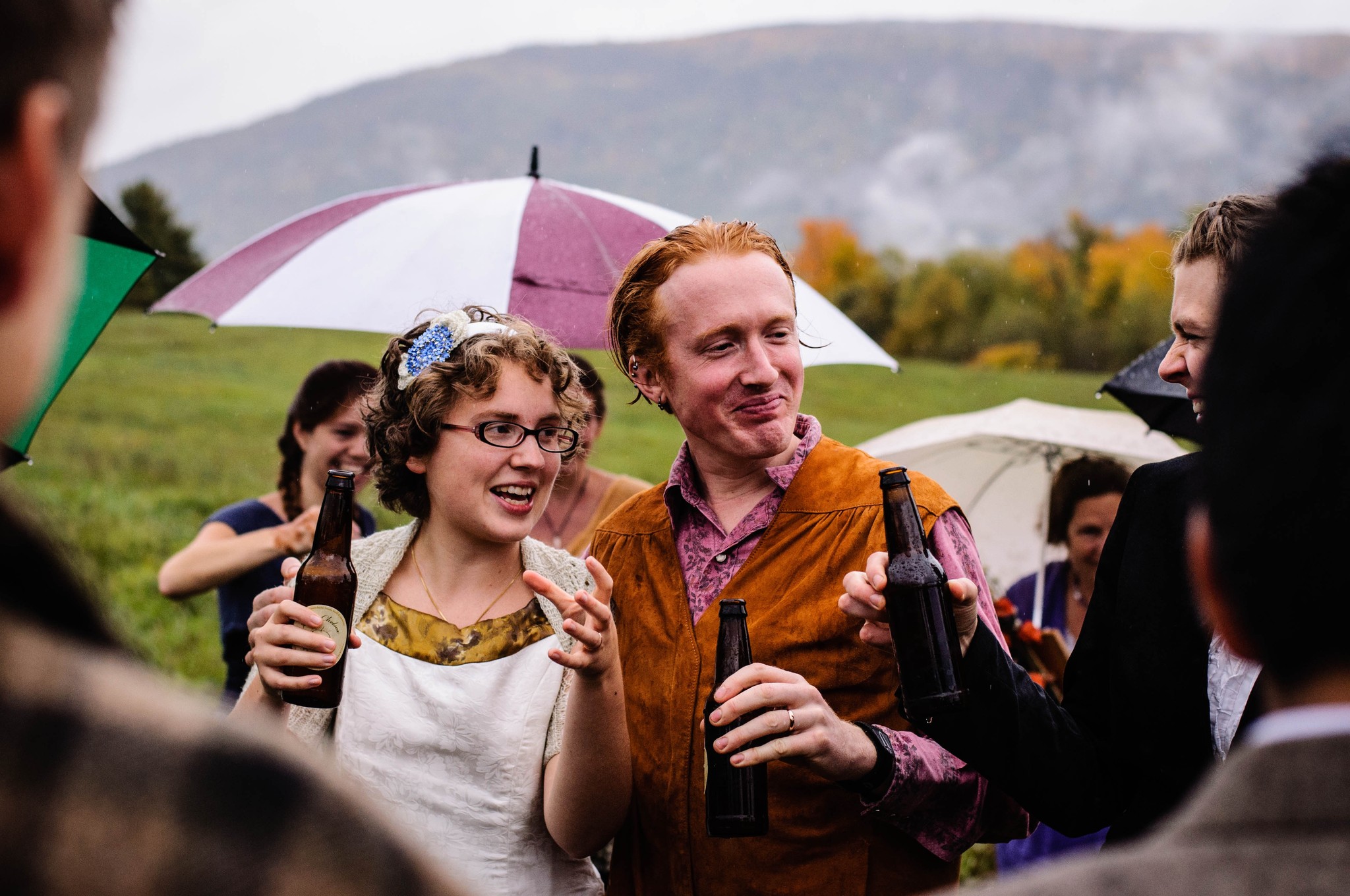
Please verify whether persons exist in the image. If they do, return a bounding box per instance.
[1,0,466,896]
[158,356,382,713]
[520,348,661,557]
[230,304,630,896]
[567,216,1019,896]
[836,146,1349,895]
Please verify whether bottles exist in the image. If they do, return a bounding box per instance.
[281,470,357,708]
[879,466,975,720]
[704,600,768,838]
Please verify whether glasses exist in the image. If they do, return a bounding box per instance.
[440,420,581,458]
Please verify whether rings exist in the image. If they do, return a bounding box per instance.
[787,708,796,731]
[585,632,603,652]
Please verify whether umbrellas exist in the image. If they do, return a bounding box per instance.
[1098,339,1199,436]
[837,397,1188,605]
[0,184,164,471]
[152,143,903,378]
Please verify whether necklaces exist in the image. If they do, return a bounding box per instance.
[541,470,592,547]
[411,546,524,636]
